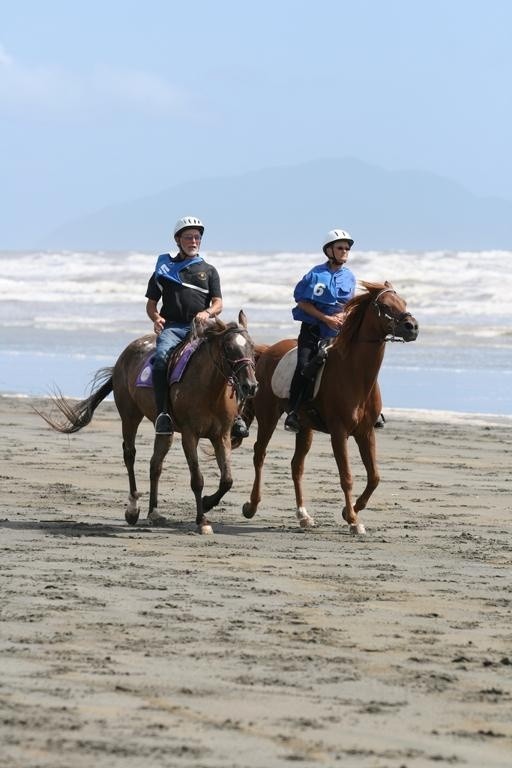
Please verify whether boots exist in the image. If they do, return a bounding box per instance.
[231,416,249,438]
[374,418,384,430]
[150,367,174,434]
[284,373,310,435]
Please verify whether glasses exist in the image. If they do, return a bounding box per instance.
[178,236,202,241]
[329,244,351,252]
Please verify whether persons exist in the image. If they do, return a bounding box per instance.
[145,215,250,438]
[284,228,386,432]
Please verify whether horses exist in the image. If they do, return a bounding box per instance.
[200,280,419,534]
[32,309,261,534]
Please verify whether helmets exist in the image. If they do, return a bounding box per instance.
[321,229,355,252]
[172,217,205,236]
